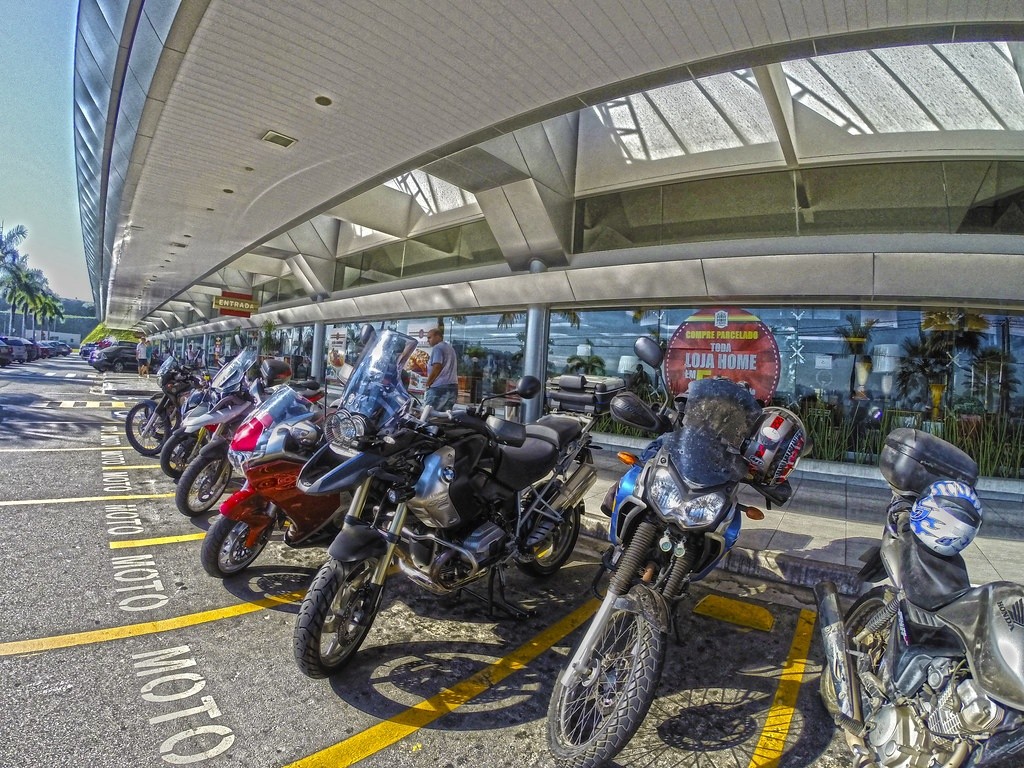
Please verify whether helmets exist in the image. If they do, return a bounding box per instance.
[740,407,807,489]
[909,480,982,560]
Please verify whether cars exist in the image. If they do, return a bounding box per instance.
[84,340,163,374]
[0,334,74,369]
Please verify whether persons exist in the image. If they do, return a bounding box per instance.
[424,328,458,410]
[185,345,202,366]
[136,338,152,378]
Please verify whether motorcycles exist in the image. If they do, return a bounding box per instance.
[293,328,629,679]
[547,334,813,768]
[811,428,1024,768]
[125,346,357,579]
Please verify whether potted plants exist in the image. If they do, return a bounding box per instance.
[896,334,950,421]
[458,362,473,393]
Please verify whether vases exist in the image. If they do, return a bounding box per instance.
[851,362,873,400]
